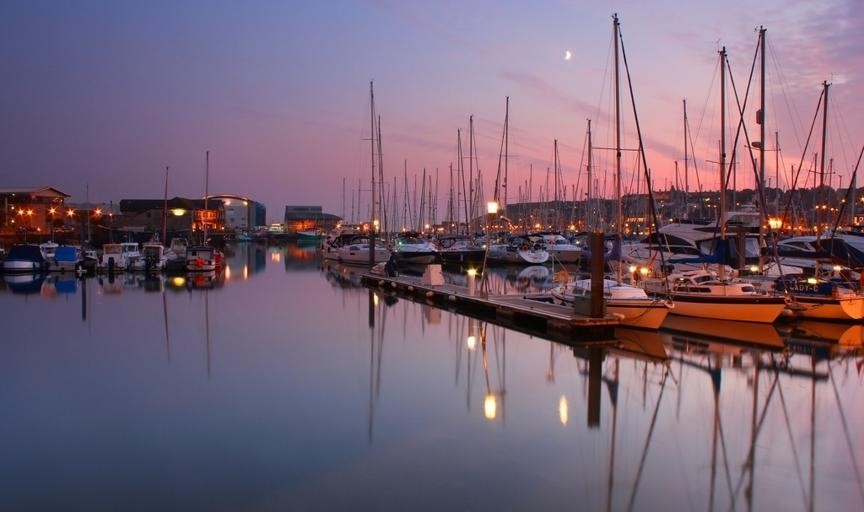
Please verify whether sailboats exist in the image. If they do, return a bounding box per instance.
[321,10,863,344]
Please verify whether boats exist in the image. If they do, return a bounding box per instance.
[0,270,225,383]
[0,150,224,272]
[236,223,321,241]
[236,238,320,249]
